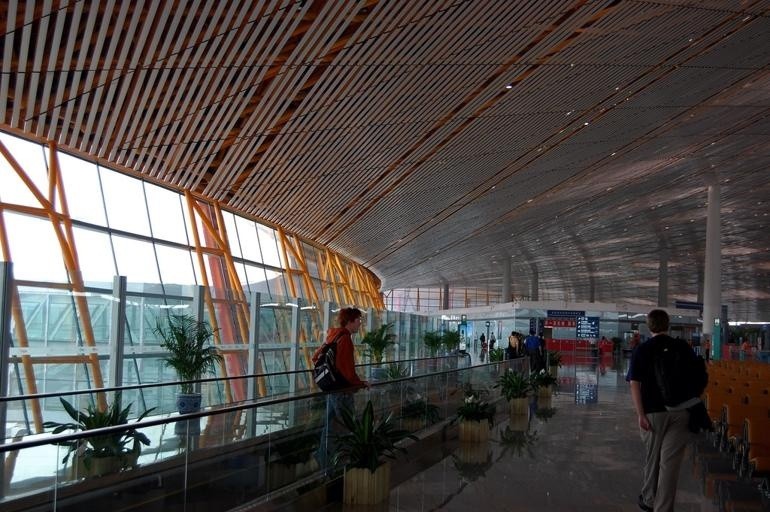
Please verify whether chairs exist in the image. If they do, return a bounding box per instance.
[693,361,770,500]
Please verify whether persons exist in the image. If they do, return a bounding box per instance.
[703,339,710,361]
[742,338,753,356]
[479,332,486,346]
[508,329,546,373]
[489,332,496,349]
[624,308,709,511]
[319,306,373,473]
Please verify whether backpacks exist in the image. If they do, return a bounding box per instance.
[310,331,352,395]
[646,334,709,410]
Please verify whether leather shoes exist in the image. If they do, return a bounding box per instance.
[638,494,654,512]
